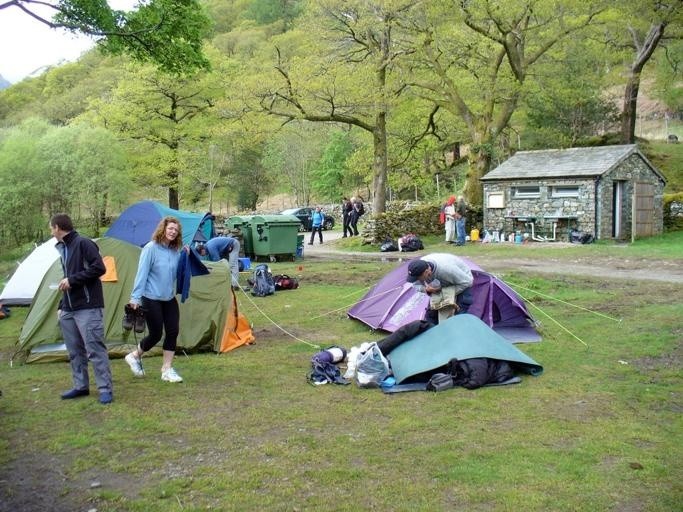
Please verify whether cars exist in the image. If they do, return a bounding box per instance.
[279,208,335,232]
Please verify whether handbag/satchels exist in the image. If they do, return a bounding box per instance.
[272,274,298,290]
[409,238,421,250]
[426,373,455,392]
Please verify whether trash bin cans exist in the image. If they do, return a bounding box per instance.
[223,215,302,263]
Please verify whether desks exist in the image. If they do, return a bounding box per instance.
[503,215,576,242]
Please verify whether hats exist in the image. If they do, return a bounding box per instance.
[406,260,429,282]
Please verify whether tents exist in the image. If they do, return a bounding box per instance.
[102,200,215,260]
[0,235,62,307]
[9,235,256,367]
[345,254,540,336]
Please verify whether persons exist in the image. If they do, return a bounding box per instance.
[307,206,324,245]
[341,197,362,238]
[195,236,241,291]
[440,195,466,247]
[124,215,191,383]
[406,252,475,325]
[50,213,114,404]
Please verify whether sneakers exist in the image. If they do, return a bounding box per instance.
[99,392,113,403]
[125,352,146,376]
[134,305,146,333]
[161,367,182,383]
[122,304,136,331]
[62,389,90,399]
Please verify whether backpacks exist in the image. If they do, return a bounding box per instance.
[253,264,276,297]
[354,203,365,217]
